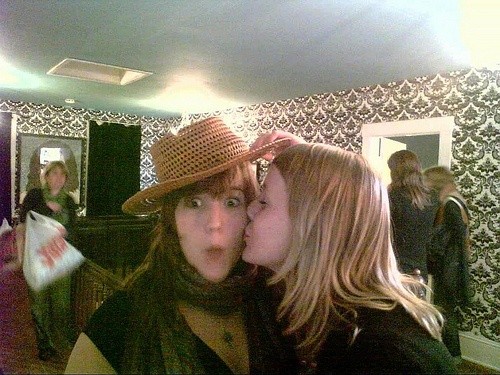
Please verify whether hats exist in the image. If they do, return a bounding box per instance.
[122,116,292,218]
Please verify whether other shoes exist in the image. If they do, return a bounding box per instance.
[64,328,77,343]
[39,347,55,361]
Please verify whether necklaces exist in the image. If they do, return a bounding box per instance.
[222,332,234,349]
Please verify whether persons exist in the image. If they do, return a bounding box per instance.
[16,161,80,361]
[387,150,471,365]
[64,116,308,375]
[243,143,460,375]
